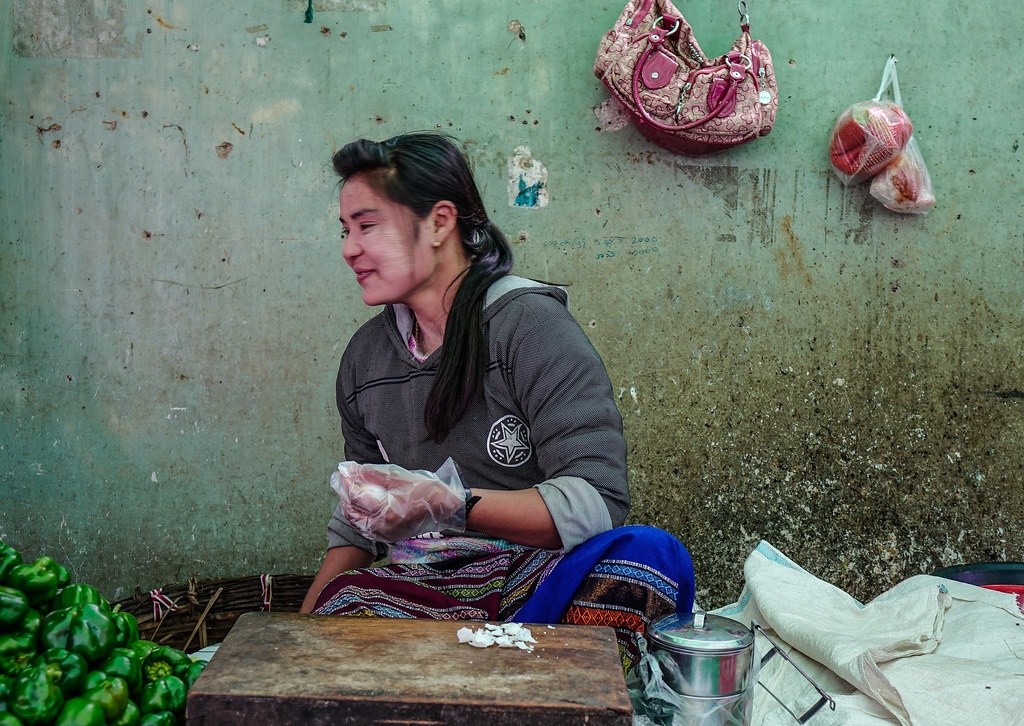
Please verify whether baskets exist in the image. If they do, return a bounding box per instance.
[108,575,316,660]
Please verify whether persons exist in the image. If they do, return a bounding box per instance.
[299,133,695,677]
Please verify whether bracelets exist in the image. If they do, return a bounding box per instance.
[448,487,482,527]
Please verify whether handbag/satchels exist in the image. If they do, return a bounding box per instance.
[594,0,778,158]
[827,57,936,214]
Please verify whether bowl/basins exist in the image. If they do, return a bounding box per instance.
[982,584,1024,612]
[929,562,1024,586]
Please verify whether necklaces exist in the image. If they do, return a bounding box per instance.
[415,322,418,343]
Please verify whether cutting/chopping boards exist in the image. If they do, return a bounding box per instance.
[185,613,634,726]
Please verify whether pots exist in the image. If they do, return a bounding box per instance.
[629,610,836,726]
